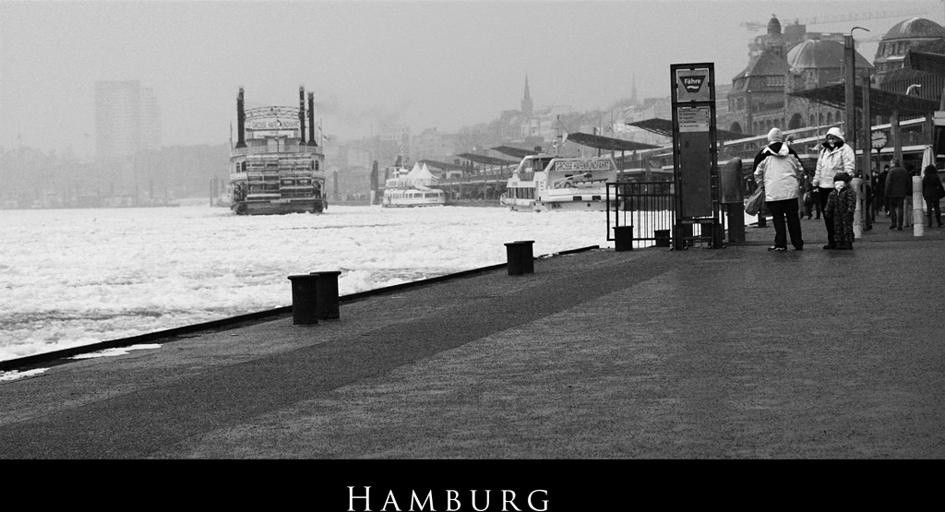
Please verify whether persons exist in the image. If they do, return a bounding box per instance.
[799,126,945,250]
[752,127,808,252]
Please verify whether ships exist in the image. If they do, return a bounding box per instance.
[224,80,328,217]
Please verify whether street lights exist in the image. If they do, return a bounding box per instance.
[844,26,875,147]
[859,63,889,181]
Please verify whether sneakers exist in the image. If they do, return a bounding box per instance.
[767,245,787,252]
[889,224,909,231]
[823,242,837,249]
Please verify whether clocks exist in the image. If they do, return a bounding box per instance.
[869,130,889,151]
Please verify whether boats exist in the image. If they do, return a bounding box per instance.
[379,188,448,208]
[498,119,628,212]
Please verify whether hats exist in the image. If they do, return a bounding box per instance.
[767,127,784,141]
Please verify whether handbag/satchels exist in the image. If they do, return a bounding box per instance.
[745,186,764,215]
